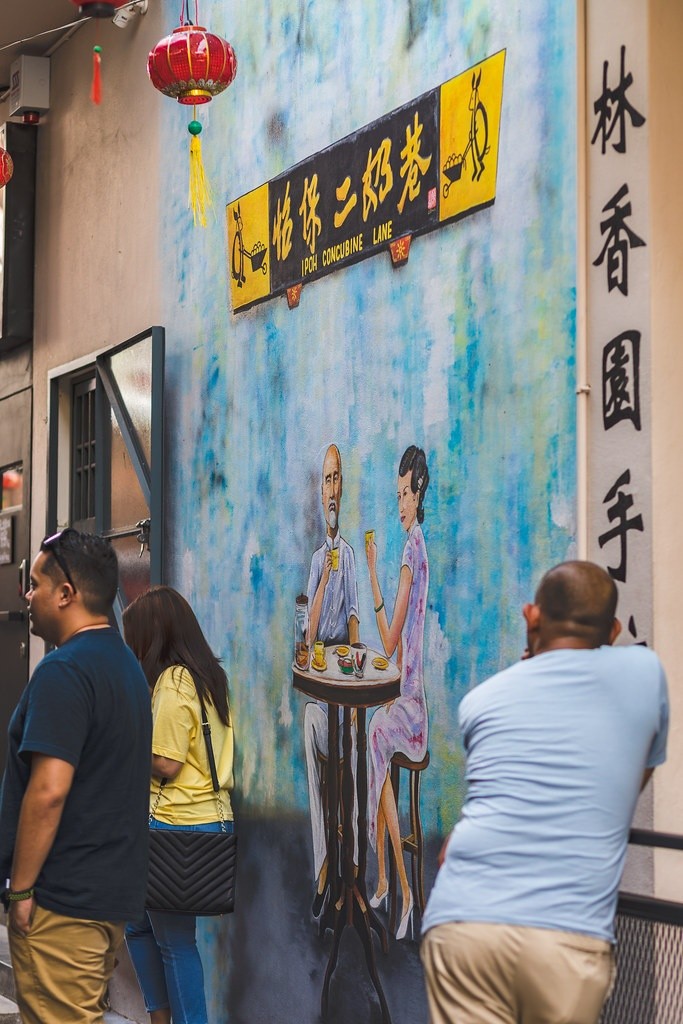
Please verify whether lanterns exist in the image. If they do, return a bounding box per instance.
[0,147,14,190]
[2,465,22,491]
[71,0,128,106]
[146,20,238,230]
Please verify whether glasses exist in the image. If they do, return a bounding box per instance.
[43,528,77,594]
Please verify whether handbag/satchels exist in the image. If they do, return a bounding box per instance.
[144,827,240,916]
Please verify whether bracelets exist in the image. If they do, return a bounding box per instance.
[5,888,35,901]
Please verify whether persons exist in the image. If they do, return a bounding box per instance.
[0,527,152,1023]
[121,583,236,1024]
[417,556,672,1024]
